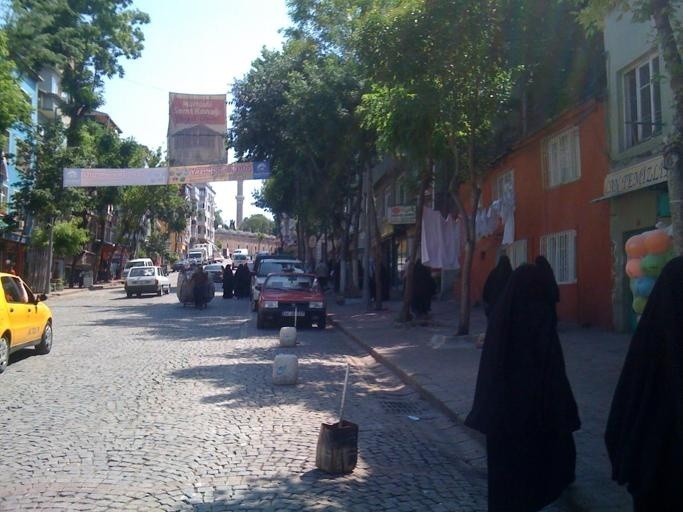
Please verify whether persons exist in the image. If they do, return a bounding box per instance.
[223,264,252,299]
[404,261,438,318]
[192,267,208,310]
[316,260,342,293]
[467,257,581,511]
[605,256,683,511]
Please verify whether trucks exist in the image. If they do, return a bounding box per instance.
[187,244,212,265]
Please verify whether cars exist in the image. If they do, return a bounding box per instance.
[253,271,325,330]
[172,260,190,271]
[0,272,53,371]
[124,265,171,297]
[123,258,153,280]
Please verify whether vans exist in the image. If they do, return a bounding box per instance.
[232,249,248,259]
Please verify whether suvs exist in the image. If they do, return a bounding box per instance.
[251,258,304,309]
